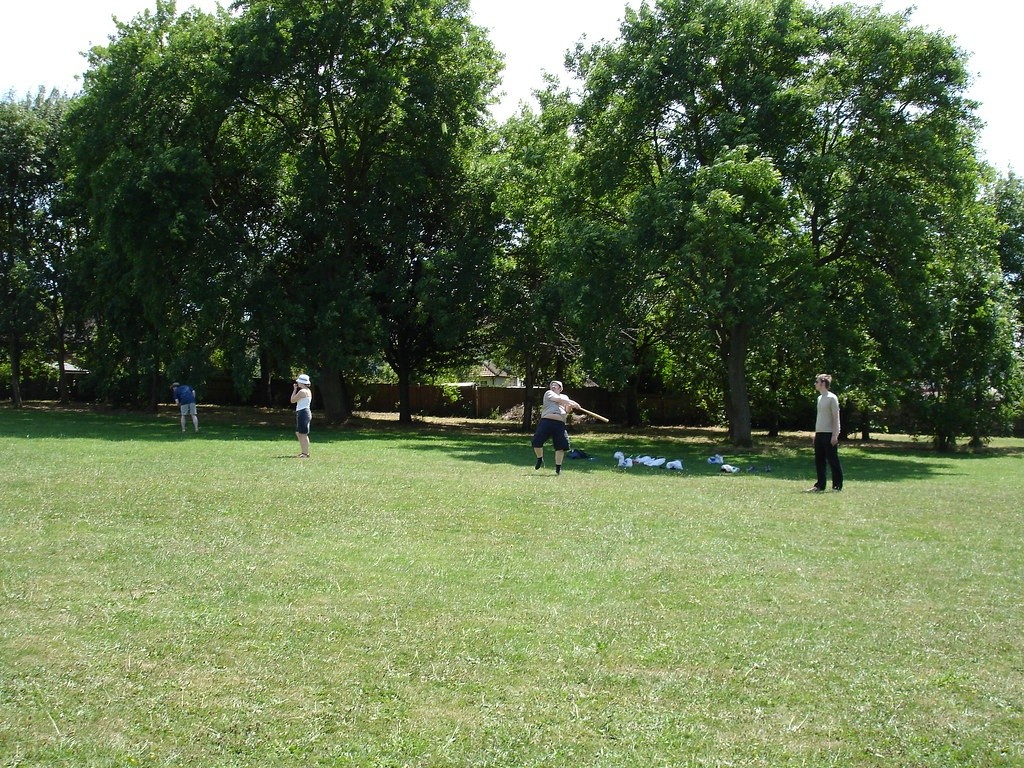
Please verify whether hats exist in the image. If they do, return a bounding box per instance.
[296,374,310,384]
[169,382,179,389]
[550,381,563,391]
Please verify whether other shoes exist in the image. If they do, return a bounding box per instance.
[535,457,544,470]
[806,486,819,492]
[296,452,310,458]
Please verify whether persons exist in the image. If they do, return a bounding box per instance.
[170,382,199,432]
[808,374,843,491]
[532,381,586,475]
[290,374,312,457]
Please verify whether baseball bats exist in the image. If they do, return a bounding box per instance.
[575,406,609,424]
